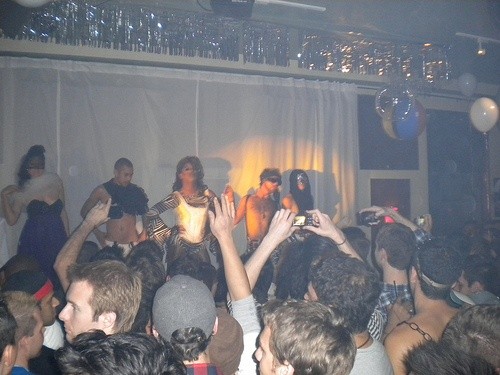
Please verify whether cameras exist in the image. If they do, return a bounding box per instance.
[108,206,123,218]
[356,211,384,225]
[292,214,314,227]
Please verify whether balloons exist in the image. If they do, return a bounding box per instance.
[471,97,499,134]
[373,83,426,144]
[14,0,49,8]
[458,71,476,99]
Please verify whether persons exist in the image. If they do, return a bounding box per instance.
[2,144,71,260]
[141,157,222,295]
[280,168,346,243]
[224,167,284,285]
[0,192,500,375]
[80,157,150,258]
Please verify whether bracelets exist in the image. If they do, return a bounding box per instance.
[334,232,347,246]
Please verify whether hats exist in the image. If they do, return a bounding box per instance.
[1,270,52,302]
[151,274,216,343]
[261,168,281,184]
